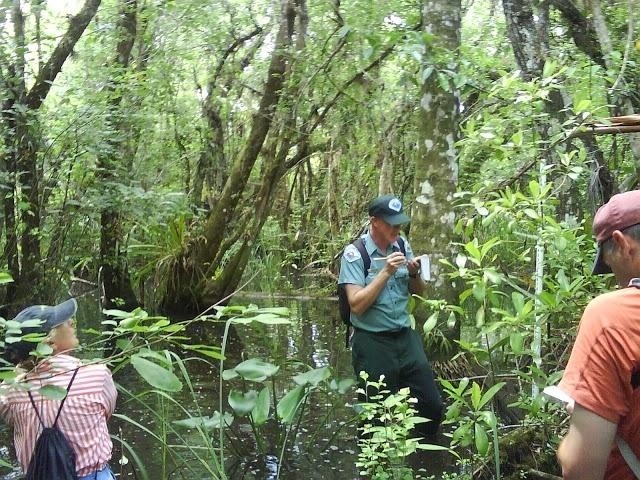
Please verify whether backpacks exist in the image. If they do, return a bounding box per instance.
[339,236,405,327]
[25,369,78,480]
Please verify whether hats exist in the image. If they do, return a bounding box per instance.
[368,195,411,226]
[591,190,640,275]
[13,299,77,333]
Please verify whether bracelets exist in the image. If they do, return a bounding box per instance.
[409,269,421,278]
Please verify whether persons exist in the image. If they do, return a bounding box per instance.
[1,299,118,479]
[556,191,640,479]
[339,195,442,443]
